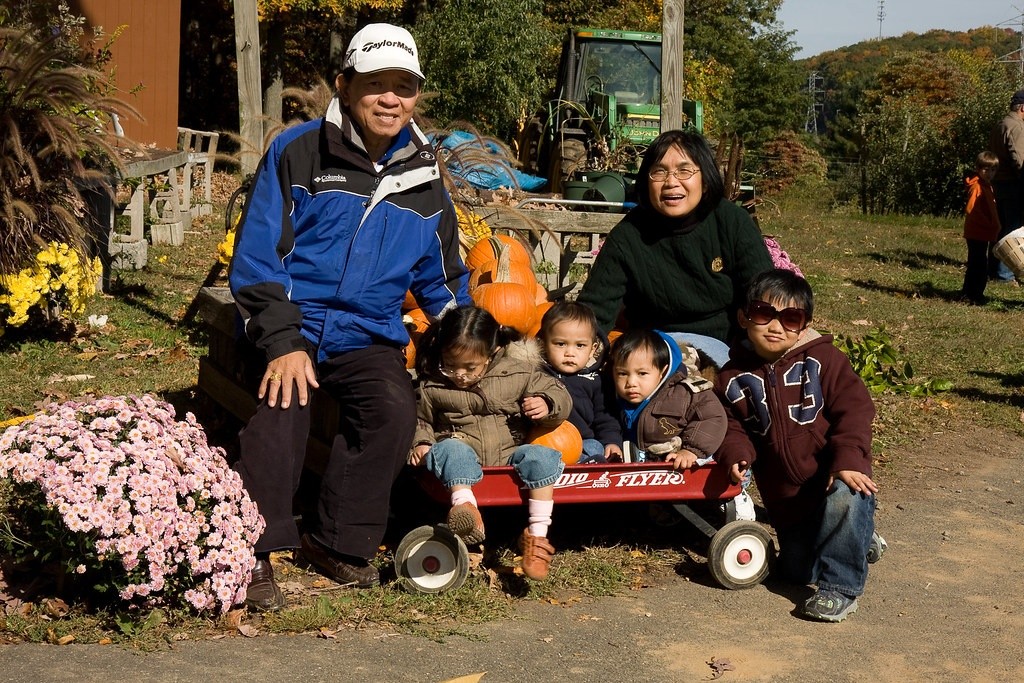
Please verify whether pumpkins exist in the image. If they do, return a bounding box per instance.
[400,234,625,370]
[524,419,583,464]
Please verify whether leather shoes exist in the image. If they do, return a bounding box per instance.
[243,555,285,612]
[292,546,380,586]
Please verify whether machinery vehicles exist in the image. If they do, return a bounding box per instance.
[520,28,703,194]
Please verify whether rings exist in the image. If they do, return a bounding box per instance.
[271,374,282,381]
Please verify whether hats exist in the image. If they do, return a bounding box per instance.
[342,23,425,80]
[1011,90,1024,104]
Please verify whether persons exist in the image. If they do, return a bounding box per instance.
[540,300,624,463]
[611,328,727,470]
[964,150,1001,304]
[713,268,887,623]
[577,130,776,370]
[227,23,474,610]
[406,306,574,581]
[988,90,1024,286]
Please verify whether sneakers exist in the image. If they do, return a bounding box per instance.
[518,528,556,581]
[803,589,859,623]
[867,531,888,564]
[447,503,486,545]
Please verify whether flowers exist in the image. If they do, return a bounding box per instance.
[3,242,102,326]
[5,394,266,617]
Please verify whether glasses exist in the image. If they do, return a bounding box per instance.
[648,167,700,182]
[746,300,807,333]
[439,359,490,385]
